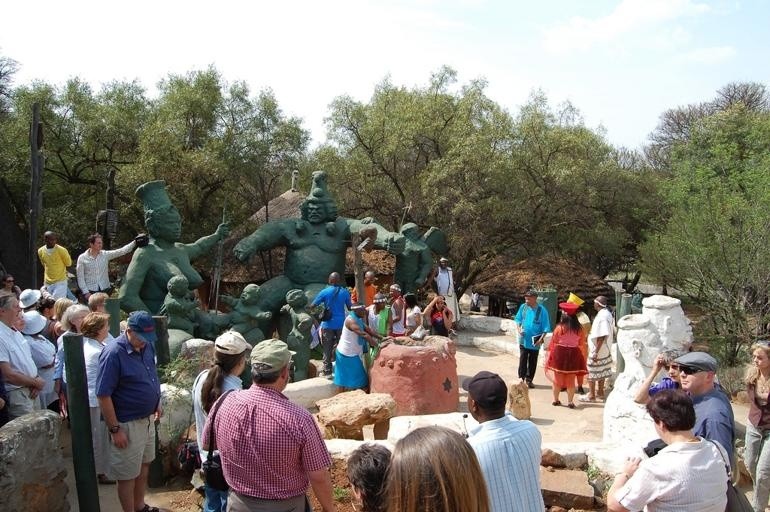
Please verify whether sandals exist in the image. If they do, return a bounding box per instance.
[141,503,160,512]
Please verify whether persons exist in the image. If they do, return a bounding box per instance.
[344,440,392,512]
[377,421,491,511]
[158,274,201,338]
[231,169,407,391]
[460,367,549,512]
[604,388,733,512]
[511,291,615,409]
[468,287,482,312]
[278,286,314,383]
[633,349,682,407]
[638,292,696,358]
[217,281,273,339]
[304,256,463,399]
[361,211,451,302]
[739,339,769,511]
[94,309,167,512]
[117,178,233,362]
[75,233,148,305]
[187,329,252,511]
[0,272,116,490]
[601,313,667,473]
[201,336,340,511]
[672,349,735,474]
[94,207,120,250]
[35,229,74,302]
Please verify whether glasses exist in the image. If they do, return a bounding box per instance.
[679,366,700,375]
[664,364,678,371]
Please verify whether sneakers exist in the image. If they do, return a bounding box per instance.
[519,378,604,408]
[325,372,332,379]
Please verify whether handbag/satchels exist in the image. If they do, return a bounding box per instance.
[201,453,226,491]
[725,480,754,512]
[318,307,333,322]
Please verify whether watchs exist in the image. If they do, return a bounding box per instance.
[108,424,120,433]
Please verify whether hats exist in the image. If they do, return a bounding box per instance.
[127,311,158,344]
[19,310,49,335]
[559,301,579,316]
[462,370,507,409]
[19,289,41,308]
[594,296,608,308]
[215,330,252,356]
[672,351,718,373]
[250,338,297,372]
[568,292,585,307]
